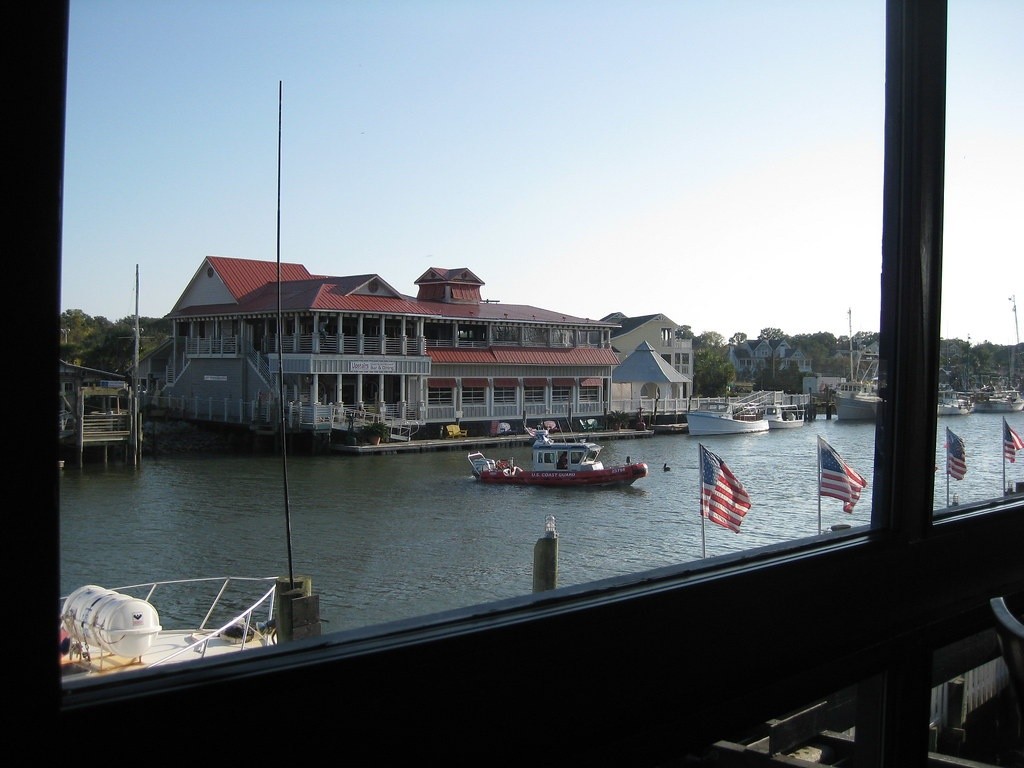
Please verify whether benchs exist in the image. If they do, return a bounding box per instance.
[500,428,518,437]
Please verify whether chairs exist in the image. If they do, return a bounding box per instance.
[445,424,467,440]
[543,421,563,433]
[578,419,605,432]
[523,426,536,438]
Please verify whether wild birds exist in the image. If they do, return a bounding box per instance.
[663,463,671,471]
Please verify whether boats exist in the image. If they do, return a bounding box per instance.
[764,406,805,428]
[834,383,877,420]
[686,402,770,436]
[468,413,647,486]
[974,391,1024,412]
[60,572,278,680]
[939,399,975,415]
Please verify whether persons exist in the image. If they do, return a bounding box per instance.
[557,451,568,470]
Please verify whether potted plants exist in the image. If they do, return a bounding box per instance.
[608,410,630,429]
[586,318,589,323]
[366,422,390,445]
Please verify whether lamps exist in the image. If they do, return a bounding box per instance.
[504,313,508,319]
[470,311,474,317]
[563,316,566,321]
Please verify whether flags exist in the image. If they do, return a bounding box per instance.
[819,439,868,514]
[700,445,751,534]
[1004,420,1024,463]
[947,429,966,481]
